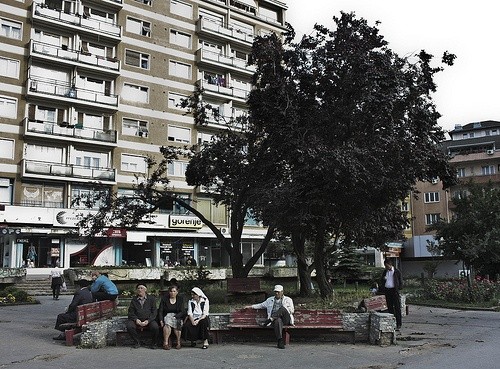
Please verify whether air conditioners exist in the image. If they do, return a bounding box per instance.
[487,149,495,155]
[221,226,229,235]
[459,269,470,277]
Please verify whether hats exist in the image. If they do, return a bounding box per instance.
[273,285,284,292]
[135,283,147,290]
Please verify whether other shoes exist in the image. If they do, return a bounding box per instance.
[151,343,158,349]
[191,343,196,346]
[203,344,209,349]
[176,344,181,349]
[135,343,141,348]
[264,318,273,327]
[163,345,170,350]
[278,340,285,349]
[53,334,65,340]
[53,297,60,300]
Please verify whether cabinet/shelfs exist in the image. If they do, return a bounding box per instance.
[3,244,10,269]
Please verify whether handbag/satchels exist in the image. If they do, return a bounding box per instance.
[62,281,67,288]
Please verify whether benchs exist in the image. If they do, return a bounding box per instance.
[116,313,229,345]
[227,277,269,304]
[364,294,388,312]
[226,307,344,346]
[58,299,119,347]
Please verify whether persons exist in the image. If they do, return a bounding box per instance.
[48,266,65,301]
[88,271,119,302]
[183,287,211,349]
[379,259,403,330]
[127,283,159,349]
[158,285,185,349]
[52,279,92,340]
[244,284,294,349]
[26,241,37,265]
[372,281,380,295]
[160,255,197,268]
